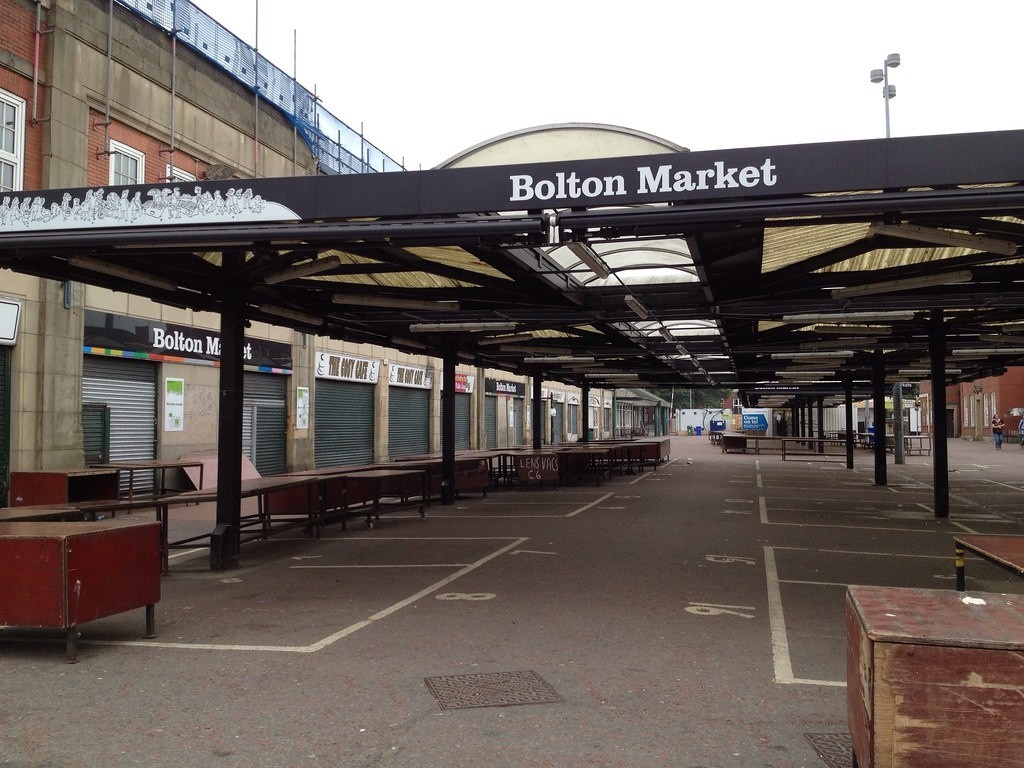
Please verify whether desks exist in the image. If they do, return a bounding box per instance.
[708,429,847,461]
[852,433,931,456]
[953,533,1024,593]
[0,439,671,665]
[846,582,1024,767]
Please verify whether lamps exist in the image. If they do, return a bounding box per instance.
[754,222,1024,408]
[66,242,721,389]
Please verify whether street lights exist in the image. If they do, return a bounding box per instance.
[870,53,901,137]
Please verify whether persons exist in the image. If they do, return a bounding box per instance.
[1018,415,1024,448]
[992,414,1005,450]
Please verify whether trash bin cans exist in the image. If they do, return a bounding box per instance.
[696,426,702,436]
[588,427,594,441]
[867,426,874,442]
[687,426,693,436]
